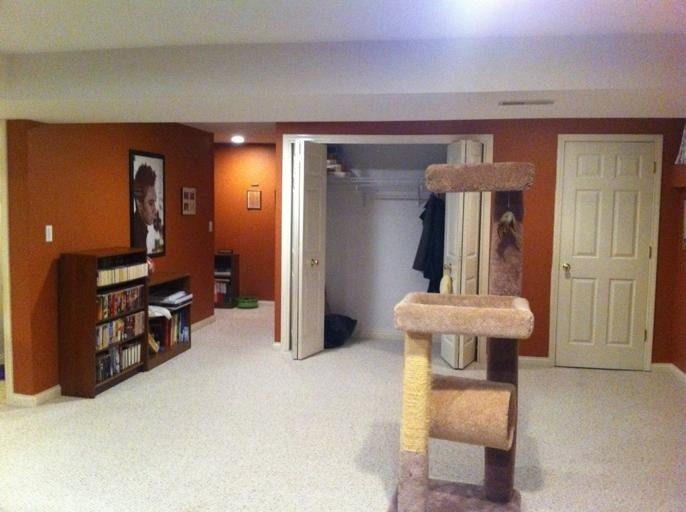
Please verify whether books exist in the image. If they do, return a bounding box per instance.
[97,260,193,386]
[214,255,231,306]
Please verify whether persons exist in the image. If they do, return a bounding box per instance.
[132,164,159,254]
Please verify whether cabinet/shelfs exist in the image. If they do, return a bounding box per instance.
[54,244,193,400]
[212,253,240,309]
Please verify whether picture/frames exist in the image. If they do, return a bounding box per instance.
[182,185,197,216]
[128,149,167,258]
